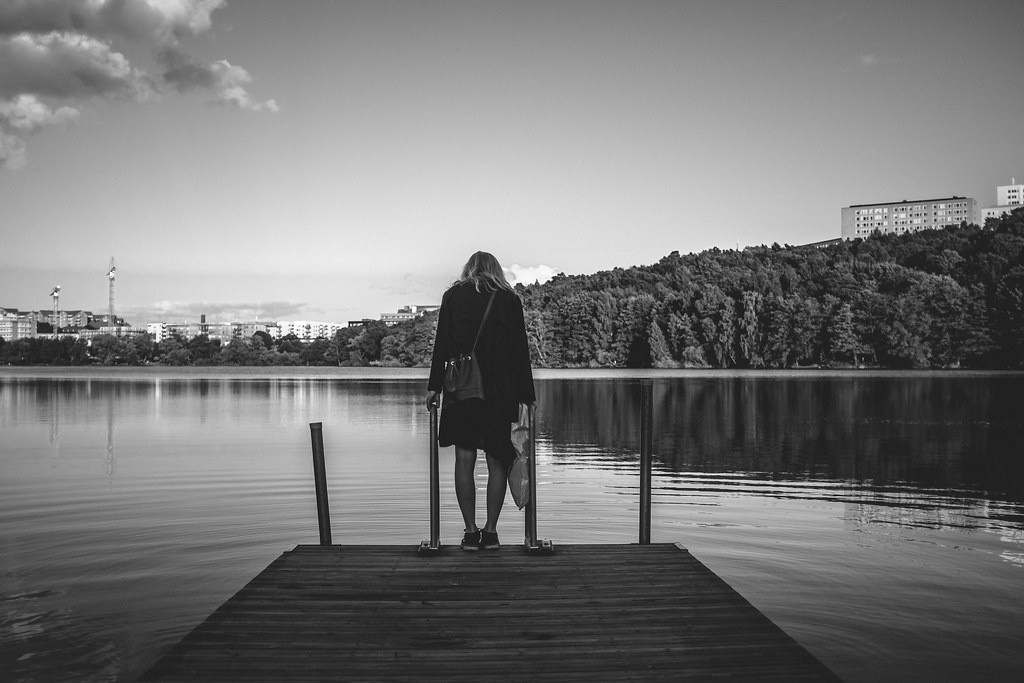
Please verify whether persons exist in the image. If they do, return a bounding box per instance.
[425,251,537,551]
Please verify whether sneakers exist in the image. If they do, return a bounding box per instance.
[460,529,480,549]
[479,529,500,548]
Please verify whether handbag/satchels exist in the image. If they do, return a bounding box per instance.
[508,403,530,510]
[442,351,485,404]
